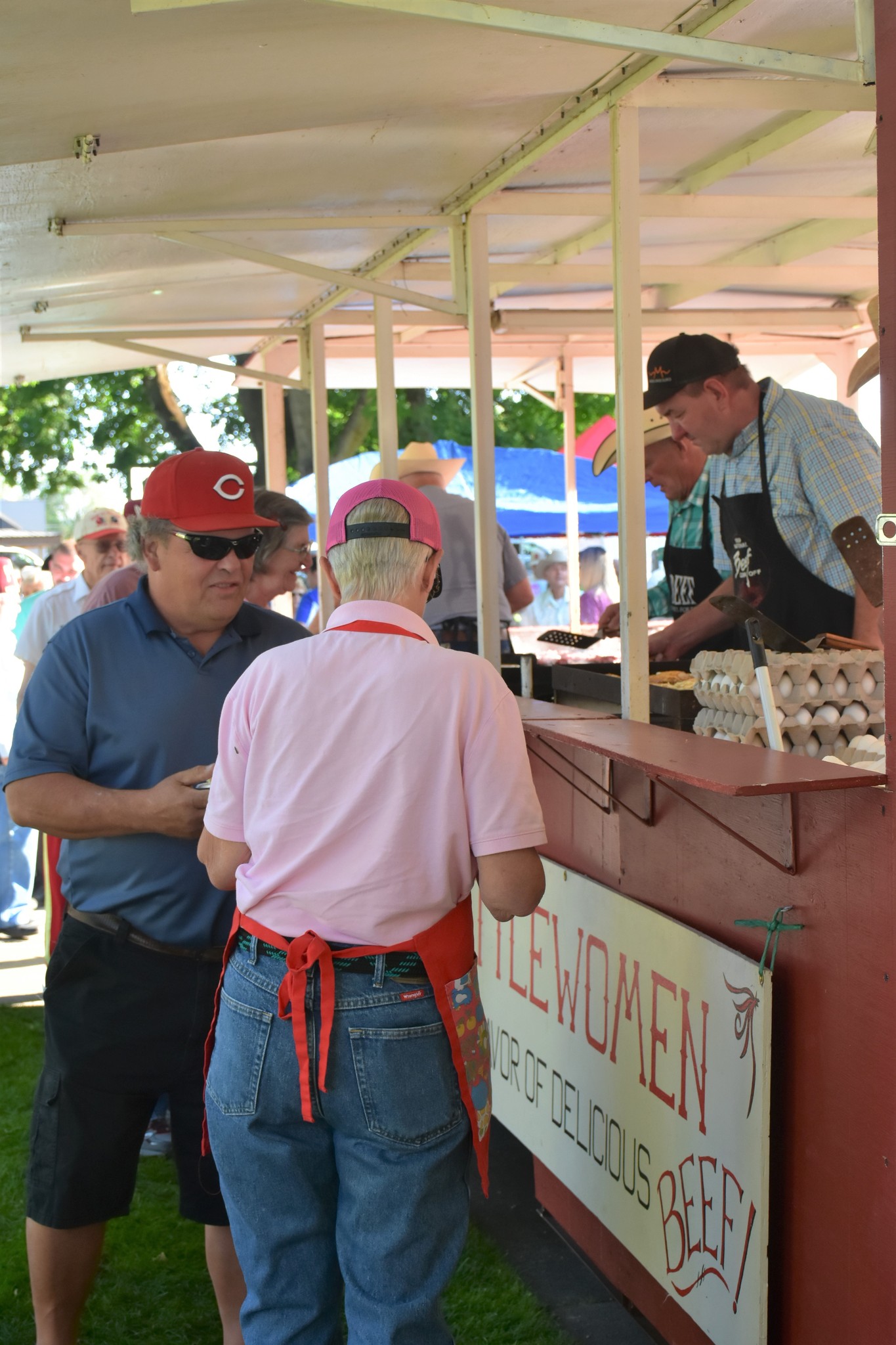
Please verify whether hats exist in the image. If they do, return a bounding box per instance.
[75,508,129,539]
[536,550,567,581]
[592,407,672,477]
[140,447,280,532]
[643,333,741,408]
[326,479,443,598]
[370,441,467,488]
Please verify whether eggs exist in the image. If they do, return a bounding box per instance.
[701,669,875,757]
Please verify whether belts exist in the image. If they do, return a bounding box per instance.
[235,933,426,978]
[67,901,226,964]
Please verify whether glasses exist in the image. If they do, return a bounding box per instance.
[279,539,313,557]
[171,528,263,560]
[81,539,129,554]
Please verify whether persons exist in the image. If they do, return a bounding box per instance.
[195,477,548,1345]
[371,440,537,655]
[645,331,884,664]
[592,408,747,662]
[0,451,313,1345]
[519,543,614,629]
[0,486,316,937]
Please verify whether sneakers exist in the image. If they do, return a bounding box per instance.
[136,1116,179,1159]
